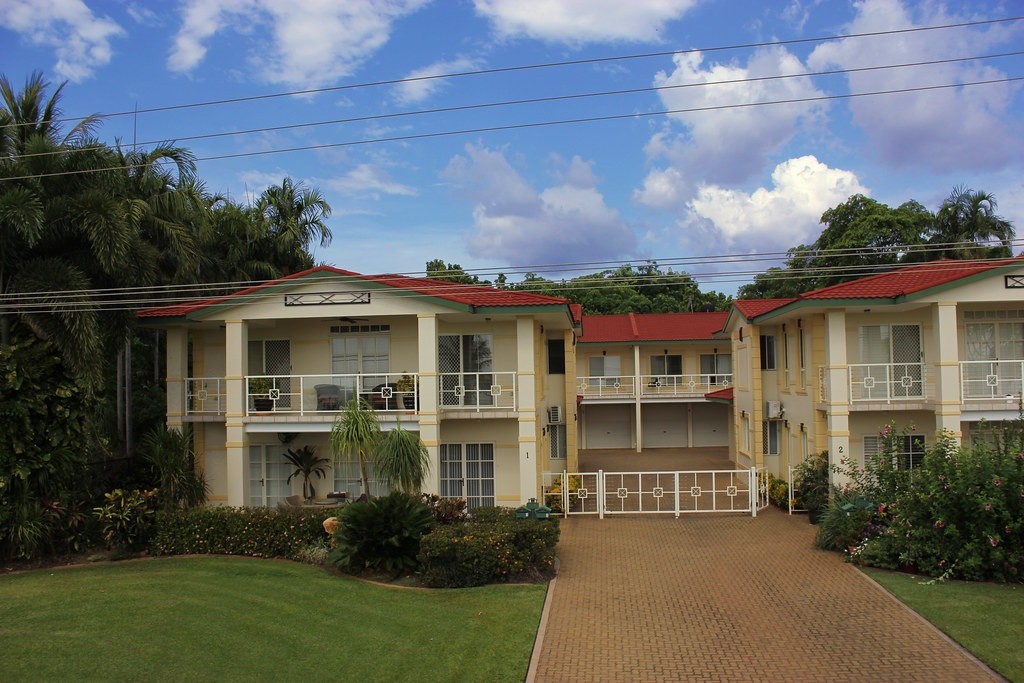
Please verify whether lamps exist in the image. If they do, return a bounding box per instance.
[800,422,807,431]
[784,420,791,429]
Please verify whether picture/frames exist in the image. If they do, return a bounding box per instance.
[1005,274,1024,288]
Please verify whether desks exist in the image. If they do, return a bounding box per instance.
[314,500,337,505]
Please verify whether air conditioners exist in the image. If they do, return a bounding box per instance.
[766,399,784,418]
[549,406,562,422]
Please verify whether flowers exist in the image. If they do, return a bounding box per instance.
[546,473,582,512]
[755,470,788,502]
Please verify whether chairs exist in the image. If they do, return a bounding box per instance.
[282,493,366,510]
[648,378,660,387]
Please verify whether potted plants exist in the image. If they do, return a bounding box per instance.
[397,371,419,411]
[248,373,281,411]
[1005,394,1014,403]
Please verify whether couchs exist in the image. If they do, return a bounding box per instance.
[371,383,398,410]
[314,383,345,410]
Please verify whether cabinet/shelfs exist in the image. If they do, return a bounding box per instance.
[327,492,349,504]
